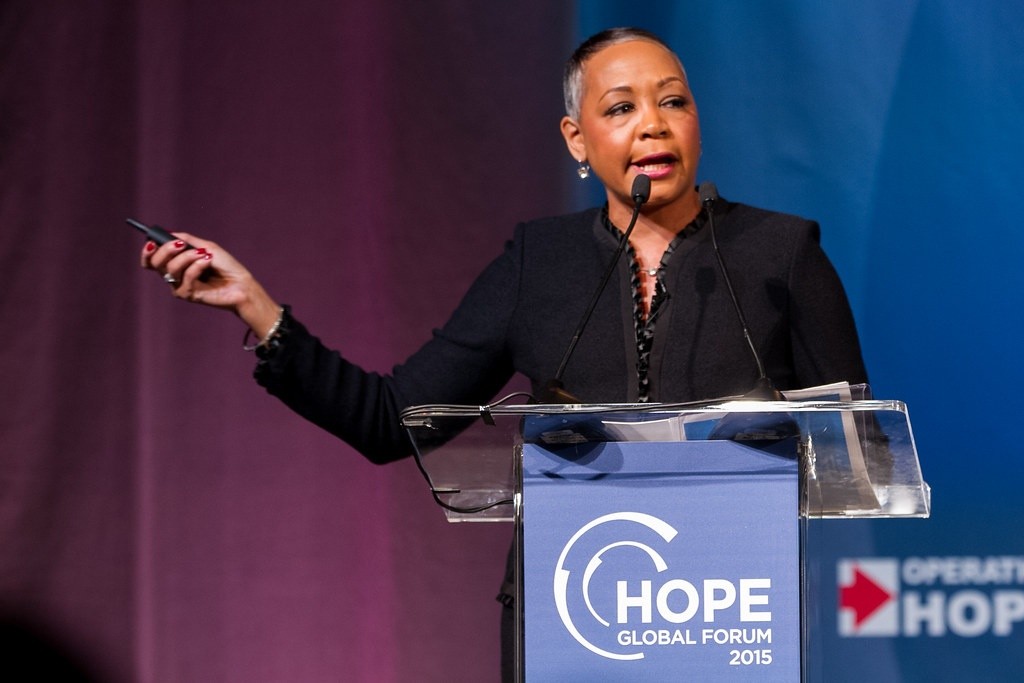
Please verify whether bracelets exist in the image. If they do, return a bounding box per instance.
[242,308,284,352]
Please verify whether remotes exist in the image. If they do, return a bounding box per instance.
[126,217,214,284]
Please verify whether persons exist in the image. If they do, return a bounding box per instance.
[139,26,894,683]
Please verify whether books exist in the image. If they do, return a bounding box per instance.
[600,381,879,514]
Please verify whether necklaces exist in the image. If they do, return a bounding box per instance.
[640,265,661,277]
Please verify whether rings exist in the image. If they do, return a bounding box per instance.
[161,271,180,285]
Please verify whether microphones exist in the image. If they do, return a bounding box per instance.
[520,174,653,445]
[697,182,802,449]
